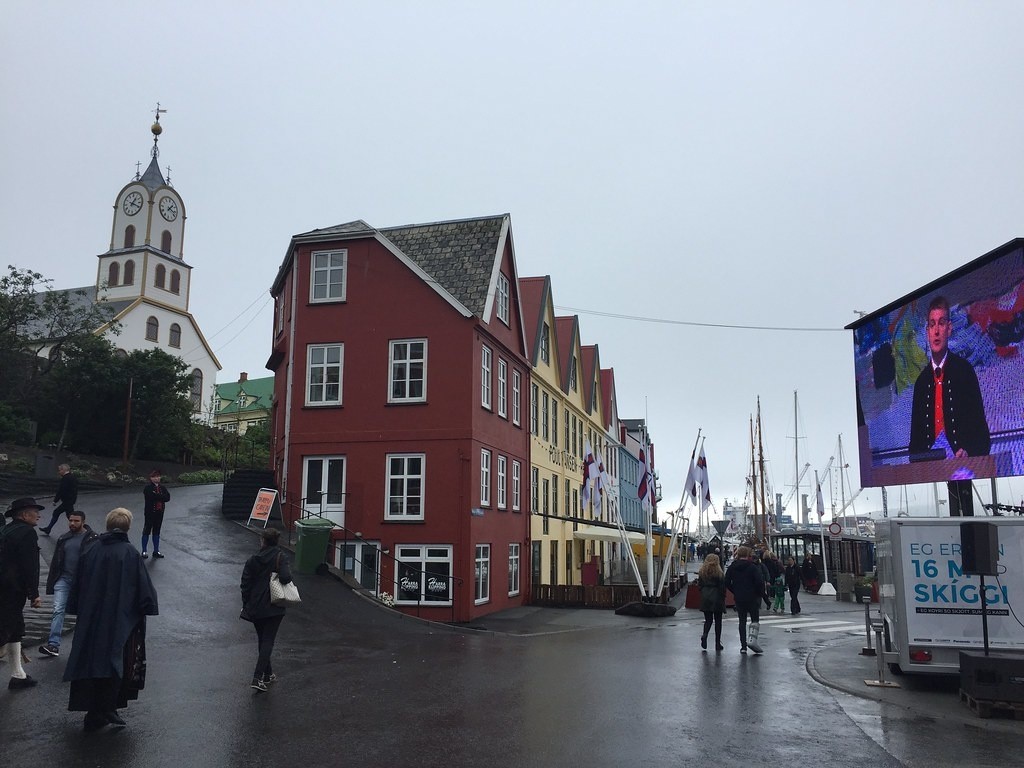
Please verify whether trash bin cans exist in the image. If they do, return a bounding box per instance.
[294,518,336,575]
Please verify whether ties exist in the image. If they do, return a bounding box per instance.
[936,367,941,377]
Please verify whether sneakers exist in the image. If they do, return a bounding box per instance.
[38,644,59,656]
[252,677,267,692]
[264,673,277,683]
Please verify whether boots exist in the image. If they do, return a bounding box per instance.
[716,641,723,650]
[701,633,707,649]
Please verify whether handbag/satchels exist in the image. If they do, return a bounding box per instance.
[270,552,302,608]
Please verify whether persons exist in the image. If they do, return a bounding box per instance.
[908,296,992,458]
[690,542,730,565]
[64,507,159,730]
[724,547,766,652]
[39,464,78,535]
[0,496,46,689]
[141,470,170,558]
[39,511,92,656]
[240,528,293,691]
[699,554,728,650]
[749,550,817,615]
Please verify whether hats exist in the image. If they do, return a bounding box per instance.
[763,550,771,559]
[4,498,45,517]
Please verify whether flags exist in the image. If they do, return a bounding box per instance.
[684,438,711,512]
[638,430,656,513]
[816,475,825,517]
[581,438,609,517]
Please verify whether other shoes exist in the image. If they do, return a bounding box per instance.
[748,642,763,654]
[83,709,126,736]
[740,647,746,653]
[792,609,798,615]
[767,602,771,610]
[8,674,38,689]
[781,609,784,613]
[773,607,777,612]
[39,527,50,534]
[152,551,164,557]
[142,552,148,558]
